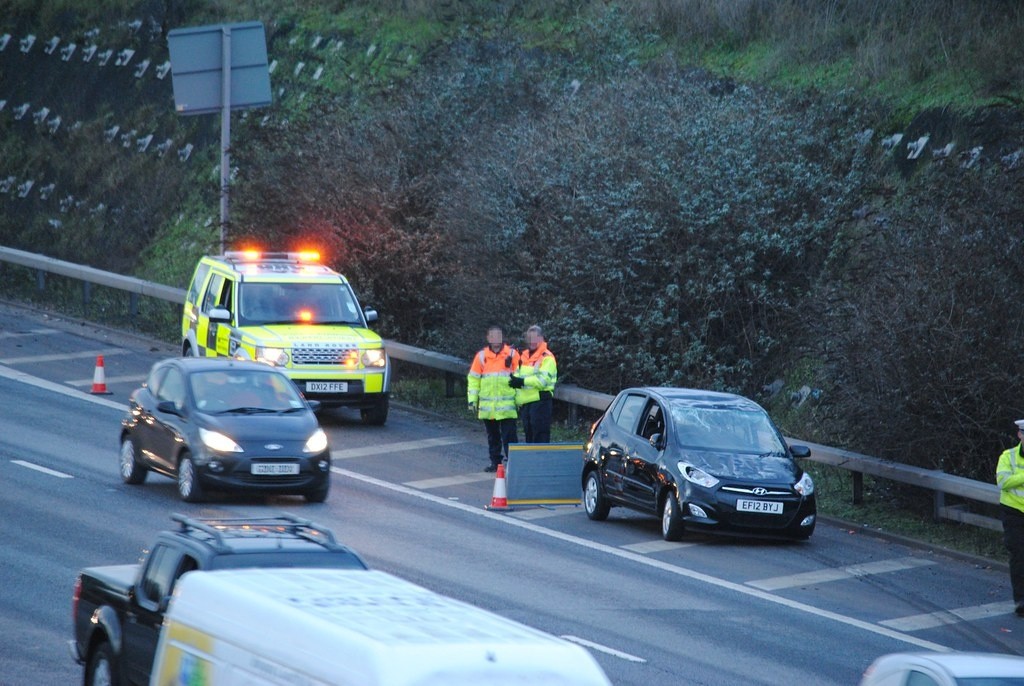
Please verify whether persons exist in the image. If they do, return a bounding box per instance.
[506,326,557,443]
[186,376,228,411]
[466,325,519,474]
[996,419,1023,619]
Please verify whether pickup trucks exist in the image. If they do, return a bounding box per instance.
[68,509,370,686]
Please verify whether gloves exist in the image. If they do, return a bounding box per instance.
[508,374,524,388]
[468,402,477,414]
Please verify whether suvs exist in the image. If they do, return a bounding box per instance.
[182,249,390,428]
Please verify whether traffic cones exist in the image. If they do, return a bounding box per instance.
[484,463,515,512]
[88,356,114,396]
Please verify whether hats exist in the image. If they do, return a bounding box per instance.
[1014,420,1024,434]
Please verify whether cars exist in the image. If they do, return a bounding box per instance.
[582,387,817,542]
[116,358,333,503]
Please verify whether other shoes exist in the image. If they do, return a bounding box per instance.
[484,460,502,472]
[1015,602,1024,615]
